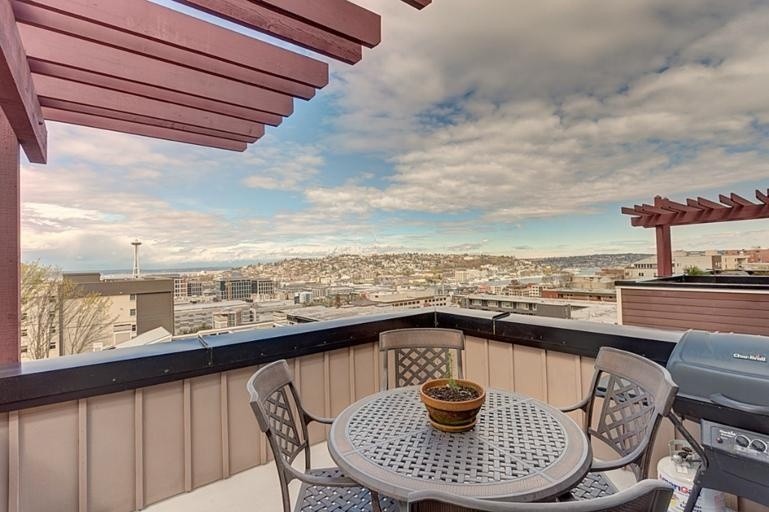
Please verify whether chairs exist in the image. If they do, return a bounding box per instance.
[380,328,465,391]
[406,479,675,511]
[562,346,678,500]
[246,359,405,512]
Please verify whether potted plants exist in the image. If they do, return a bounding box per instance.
[421,351,486,431]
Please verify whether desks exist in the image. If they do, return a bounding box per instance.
[327,385,593,511]
